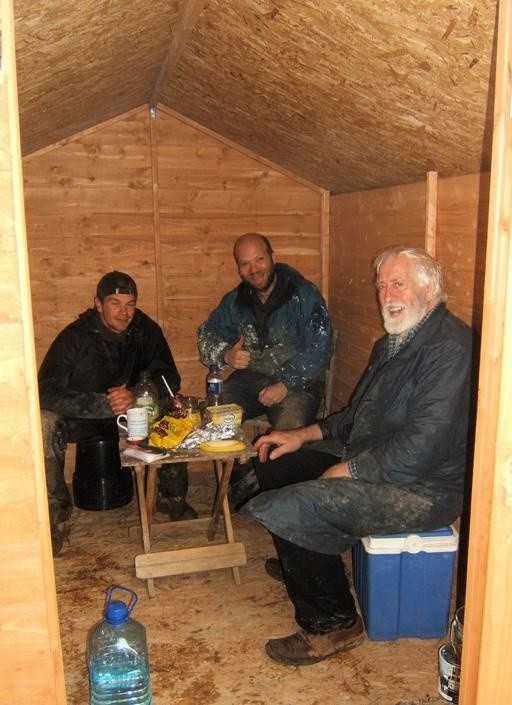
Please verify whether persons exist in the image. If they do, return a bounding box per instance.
[251,246,475,666]
[196,233,334,517]
[37,270,199,558]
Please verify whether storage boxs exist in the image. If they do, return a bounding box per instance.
[352,521,458,641]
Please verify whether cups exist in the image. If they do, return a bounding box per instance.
[116,407,149,439]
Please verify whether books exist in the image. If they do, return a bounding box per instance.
[122,441,171,465]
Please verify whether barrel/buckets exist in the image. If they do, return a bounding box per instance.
[450,606,465,662]
[437,640,462,703]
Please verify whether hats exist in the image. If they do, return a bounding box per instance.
[93,272,138,296]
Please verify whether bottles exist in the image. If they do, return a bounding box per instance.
[205,365,226,411]
[133,372,160,424]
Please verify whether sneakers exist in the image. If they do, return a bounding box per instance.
[265,557,355,588]
[156,488,198,522]
[264,616,367,666]
